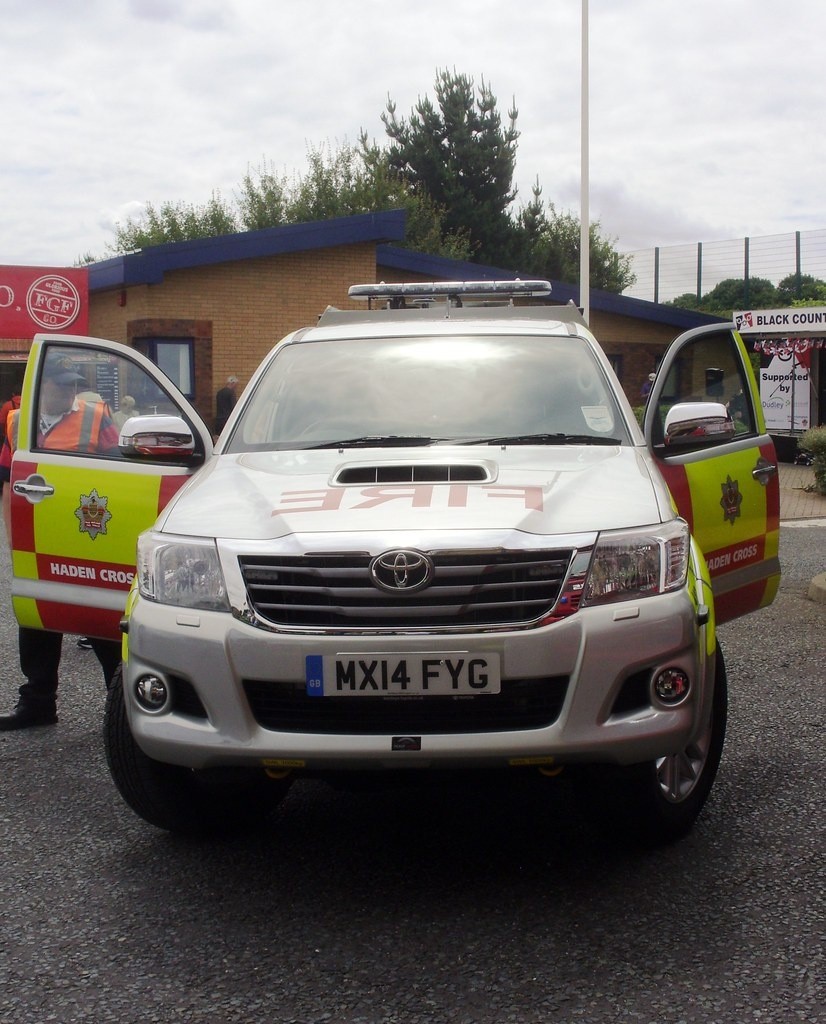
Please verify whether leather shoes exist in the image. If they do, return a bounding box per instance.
[0,696,56,728]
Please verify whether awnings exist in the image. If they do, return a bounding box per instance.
[0,352,117,365]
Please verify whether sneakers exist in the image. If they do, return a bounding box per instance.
[77,637,93,649]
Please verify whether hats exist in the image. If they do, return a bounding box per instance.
[42,351,87,386]
[648,373,655,381]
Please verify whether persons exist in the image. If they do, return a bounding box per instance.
[216,376,239,436]
[640,373,661,406]
[113,394,140,434]
[0,352,125,733]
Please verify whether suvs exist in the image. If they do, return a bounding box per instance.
[10,276,783,854]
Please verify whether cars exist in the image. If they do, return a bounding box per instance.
[793,453,814,466]
